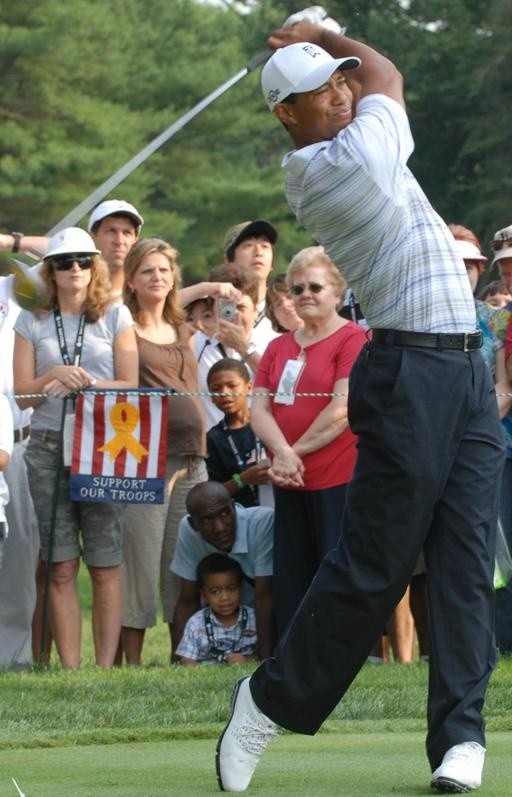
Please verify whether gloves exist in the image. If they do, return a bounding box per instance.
[282,2,353,37]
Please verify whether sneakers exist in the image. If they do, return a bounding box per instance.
[217,672,279,792]
[426,740,501,792]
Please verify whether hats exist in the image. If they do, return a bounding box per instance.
[490,222,511,270]
[88,199,143,232]
[44,227,104,262]
[456,237,488,266]
[255,41,364,109]
[226,218,280,250]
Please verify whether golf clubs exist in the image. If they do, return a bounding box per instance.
[10,46,276,310]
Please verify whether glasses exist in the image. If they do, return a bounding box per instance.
[289,281,328,297]
[52,257,100,269]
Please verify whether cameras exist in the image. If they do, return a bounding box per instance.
[218,298,237,322]
[216,648,233,666]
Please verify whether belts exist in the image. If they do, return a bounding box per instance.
[365,324,485,354]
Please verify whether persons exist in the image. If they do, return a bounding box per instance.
[213,18,505,797]
[1,198,512,668]
